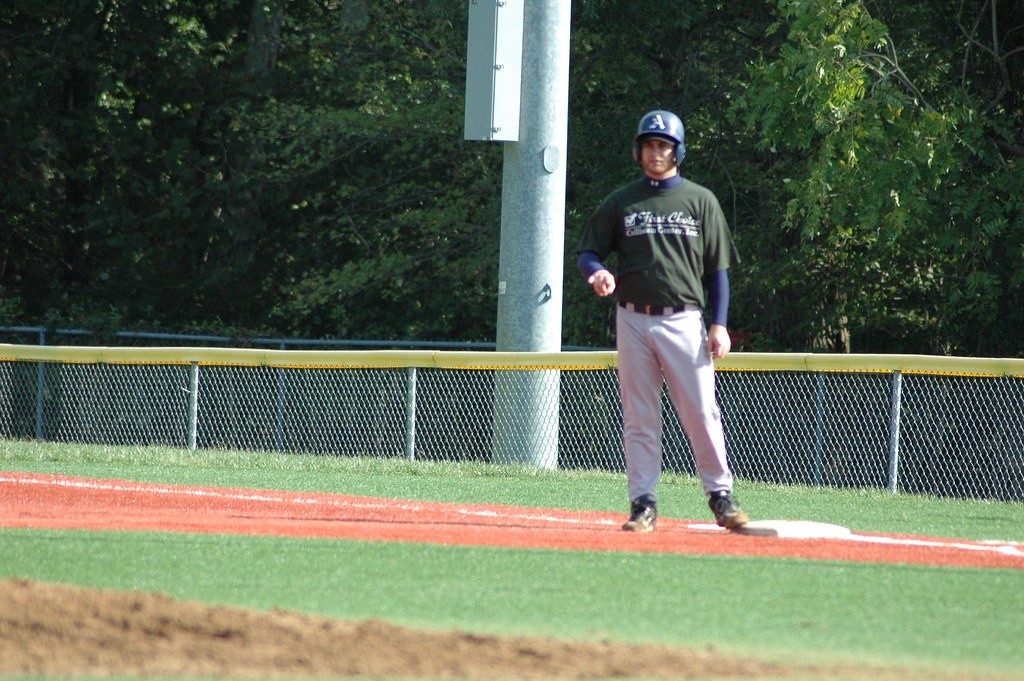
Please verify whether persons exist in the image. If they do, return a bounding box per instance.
[576,111,750,533]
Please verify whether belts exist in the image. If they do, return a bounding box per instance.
[619,301,684,316]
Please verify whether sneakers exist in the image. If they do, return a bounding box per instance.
[623,499,657,532]
[708,491,748,528]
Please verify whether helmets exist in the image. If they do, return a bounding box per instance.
[633,110,686,166]
